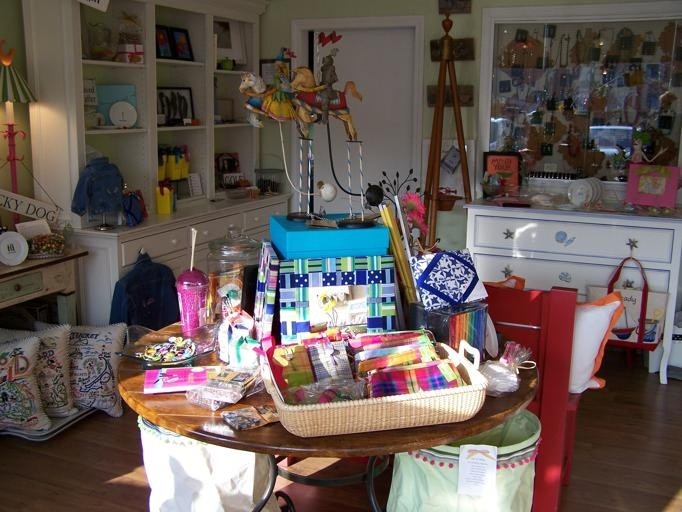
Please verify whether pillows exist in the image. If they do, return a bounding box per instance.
[0,317,128,432]
[482,275,625,393]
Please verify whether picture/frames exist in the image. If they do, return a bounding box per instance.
[157,86,195,127]
[213,16,247,65]
[154,25,195,60]
[214,153,240,174]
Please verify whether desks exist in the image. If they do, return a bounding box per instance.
[0,246,89,325]
[116,322,539,511]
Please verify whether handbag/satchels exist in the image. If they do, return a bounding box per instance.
[586,256,669,350]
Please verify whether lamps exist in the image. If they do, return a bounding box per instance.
[1,40,39,228]
[71,157,124,233]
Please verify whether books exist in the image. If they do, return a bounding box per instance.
[141,367,209,395]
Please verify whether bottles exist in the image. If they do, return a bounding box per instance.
[207,225,261,324]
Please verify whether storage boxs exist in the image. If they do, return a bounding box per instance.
[157,155,190,217]
[247,213,395,345]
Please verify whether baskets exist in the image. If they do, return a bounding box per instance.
[253,331,489,438]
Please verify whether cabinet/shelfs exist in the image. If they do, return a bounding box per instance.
[464,198,680,385]
[22,1,292,326]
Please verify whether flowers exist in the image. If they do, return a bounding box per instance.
[402,194,431,238]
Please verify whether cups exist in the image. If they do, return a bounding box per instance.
[175,267,209,340]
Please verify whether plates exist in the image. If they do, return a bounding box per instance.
[484,314,499,359]
[567,177,602,207]
[0,231,29,266]
[556,203,578,211]
[95,101,138,129]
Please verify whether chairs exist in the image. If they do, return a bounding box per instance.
[476,284,583,511]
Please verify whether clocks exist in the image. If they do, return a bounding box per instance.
[108,101,137,129]
